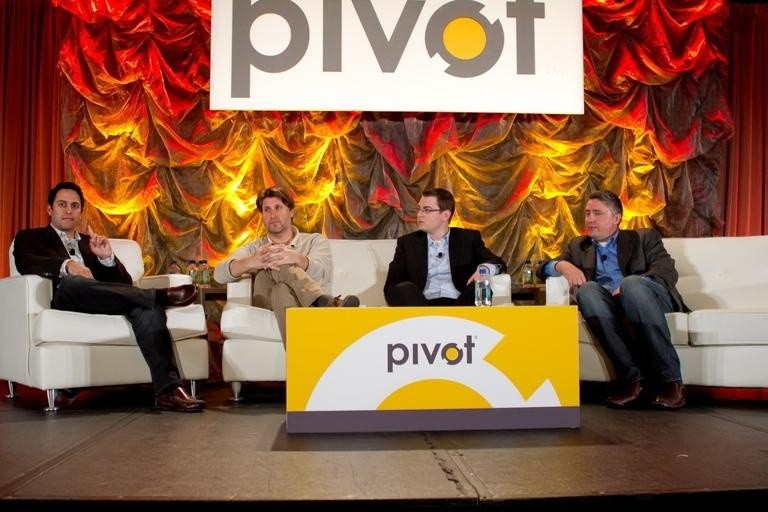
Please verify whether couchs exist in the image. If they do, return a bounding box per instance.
[220,234,514,405]
[543,234,767,410]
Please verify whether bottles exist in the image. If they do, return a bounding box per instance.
[523,259,533,284]
[168,259,180,274]
[474,269,491,306]
[196,260,211,289]
[185,259,198,288]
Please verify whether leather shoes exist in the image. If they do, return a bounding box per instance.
[605,381,643,410]
[163,284,199,306]
[153,387,206,412]
[652,380,688,408]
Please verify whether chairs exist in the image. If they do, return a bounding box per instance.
[1,232,213,412]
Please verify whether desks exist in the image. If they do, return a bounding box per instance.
[511,285,548,305]
[281,304,582,435]
[185,285,223,307]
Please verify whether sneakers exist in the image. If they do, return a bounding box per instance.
[328,294,360,306]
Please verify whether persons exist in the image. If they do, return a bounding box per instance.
[214,185,360,353]
[535,189,693,409]
[384,187,507,306]
[13,182,207,412]
